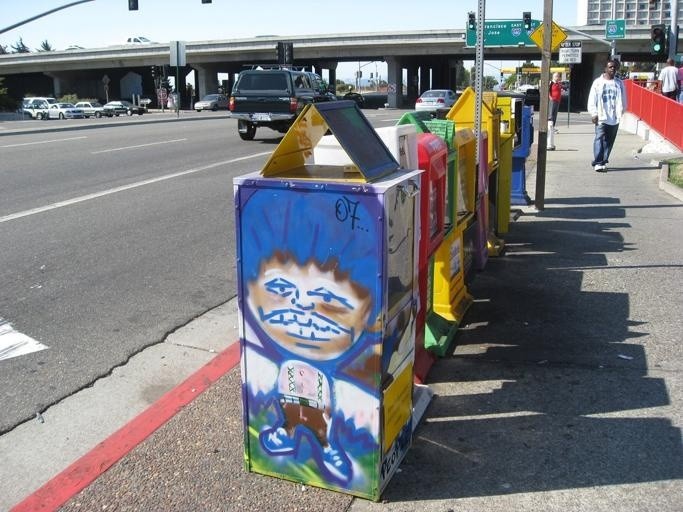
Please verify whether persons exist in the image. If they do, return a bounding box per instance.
[678,64,683,100]
[656,58,680,100]
[586,60,626,173]
[547,73,564,136]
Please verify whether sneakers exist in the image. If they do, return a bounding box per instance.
[603,165,608,171]
[595,165,603,171]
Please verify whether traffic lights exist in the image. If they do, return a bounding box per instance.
[501,72,503,78]
[150,65,156,78]
[650,23,666,56]
[468,12,475,30]
[522,12,531,31]
[164,64,169,77]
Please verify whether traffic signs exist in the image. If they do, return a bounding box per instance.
[465,20,540,48]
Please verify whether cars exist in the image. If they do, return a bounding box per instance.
[414,89,459,114]
[19,96,147,119]
[193,94,228,112]
[523,89,539,104]
[124,36,157,45]
[343,93,366,110]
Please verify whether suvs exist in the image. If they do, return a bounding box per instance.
[227,66,339,140]
